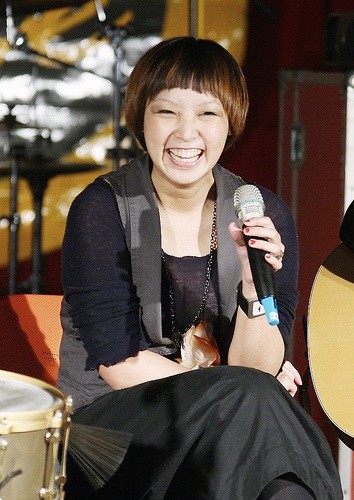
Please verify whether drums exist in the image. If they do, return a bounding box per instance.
[0,369,73,500]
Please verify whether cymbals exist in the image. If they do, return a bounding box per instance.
[0,117,48,136]
[0,162,103,182]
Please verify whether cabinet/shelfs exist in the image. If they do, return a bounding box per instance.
[217,69,346,468]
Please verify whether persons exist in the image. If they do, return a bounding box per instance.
[58,37,344,500]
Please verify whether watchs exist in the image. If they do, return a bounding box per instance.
[236,280,267,320]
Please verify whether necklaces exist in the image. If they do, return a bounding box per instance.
[160,193,217,348]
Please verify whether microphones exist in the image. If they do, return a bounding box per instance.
[234,185,279,325]
